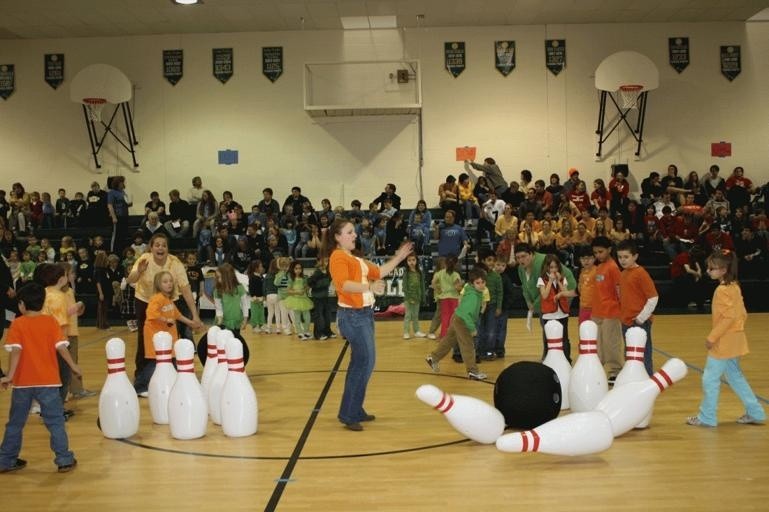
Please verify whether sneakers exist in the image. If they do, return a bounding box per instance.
[252,325,337,340]
[403,331,505,381]
[737,414,755,424]
[38,389,97,424]
[358,408,375,421]
[608,376,616,384]
[126,319,135,332]
[0,459,27,473]
[133,320,138,331]
[687,416,701,426]
[58,459,76,472]
[338,414,363,431]
[137,391,148,398]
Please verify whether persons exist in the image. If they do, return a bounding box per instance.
[133,269,195,399]
[319,219,414,432]
[0,250,96,473]
[535,253,571,364]
[0,156,768,360]
[615,240,660,379]
[125,232,204,379]
[685,247,766,428]
[588,234,622,384]
[424,268,490,379]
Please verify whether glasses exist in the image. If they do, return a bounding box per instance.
[706,267,721,272]
